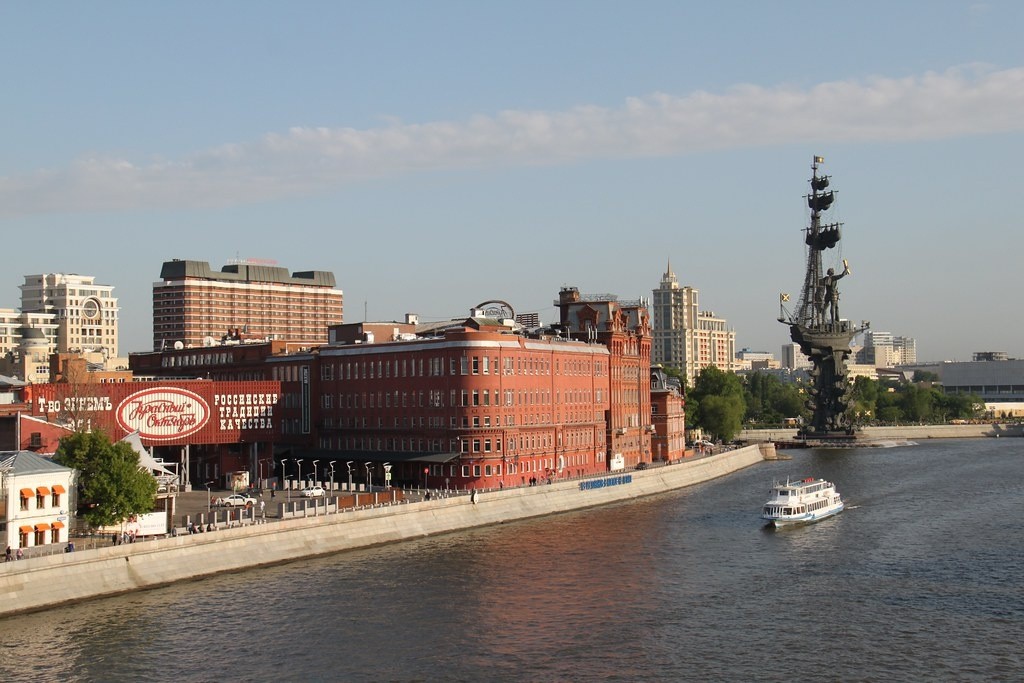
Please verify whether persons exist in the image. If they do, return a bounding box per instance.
[16,547,23,560]
[112,530,136,546]
[270,489,275,501]
[260,499,265,512]
[824,266,849,324]
[6,546,11,561]
[68,541,74,552]
[529,476,536,486]
[172,525,177,537]
[188,521,212,534]
[500,480,503,490]
[210,495,224,507]
[244,504,250,515]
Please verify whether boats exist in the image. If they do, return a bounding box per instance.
[759,476,845,528]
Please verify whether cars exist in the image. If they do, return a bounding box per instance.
[299,486,326,499]
[221,495,257,507]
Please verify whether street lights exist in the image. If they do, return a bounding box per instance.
[348,469,355,495]
[313,460,319,486]
[307,473,315,508]
[297,459,304,491]
[285,475,293,511]
[205,481,214,513]
[281,459,288,491]
[258,459,266,481]
[330,461,336,490]
[328,471,336,504]
[365,462,371,492]
[368,467,375,493]
[347,461,353,492]
[383,462,389,490]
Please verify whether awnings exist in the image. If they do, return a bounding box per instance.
[51,522,64,530]
[20,488,35,498]
[19,526,34,534]
[36,487,50,496]
[52,485,65,494]
[34,524,50,533]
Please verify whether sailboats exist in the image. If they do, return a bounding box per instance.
[776,154,869,351]
